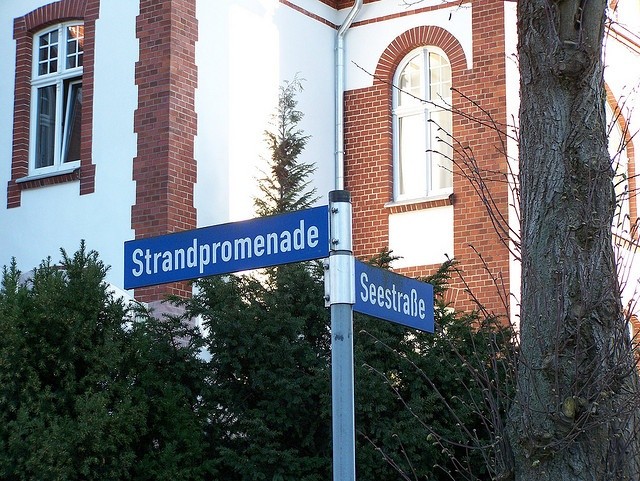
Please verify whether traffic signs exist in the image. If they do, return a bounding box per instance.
[123,205,329,290]
[353,257,435,335]
